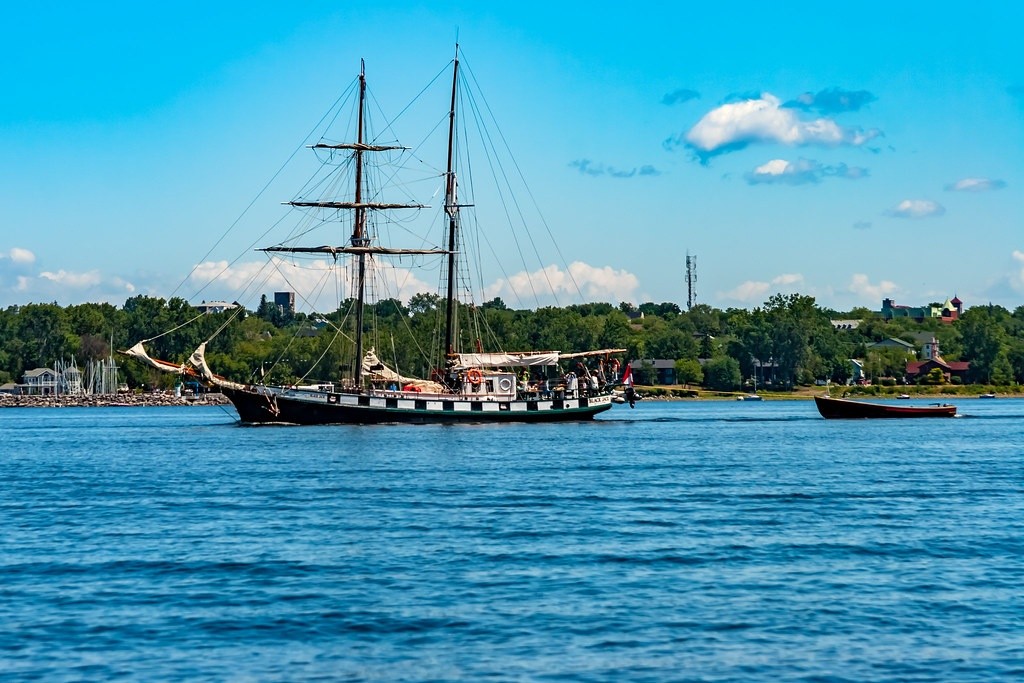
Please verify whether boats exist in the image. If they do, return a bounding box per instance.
[896,395,910,399]
[979,394,995,398]
[744,395,762,401]
[814,394,957,419]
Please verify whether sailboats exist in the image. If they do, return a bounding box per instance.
[116,41,626,426]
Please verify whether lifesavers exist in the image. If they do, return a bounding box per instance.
[500,378,511,391]
[467,369,481,385]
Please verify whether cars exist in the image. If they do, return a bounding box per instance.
[849,381,856,386]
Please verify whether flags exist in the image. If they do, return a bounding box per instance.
[623,363,634,388]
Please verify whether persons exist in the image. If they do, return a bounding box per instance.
[520,357,619,397]
[390,383,397,390]
[456,370,465,392]
